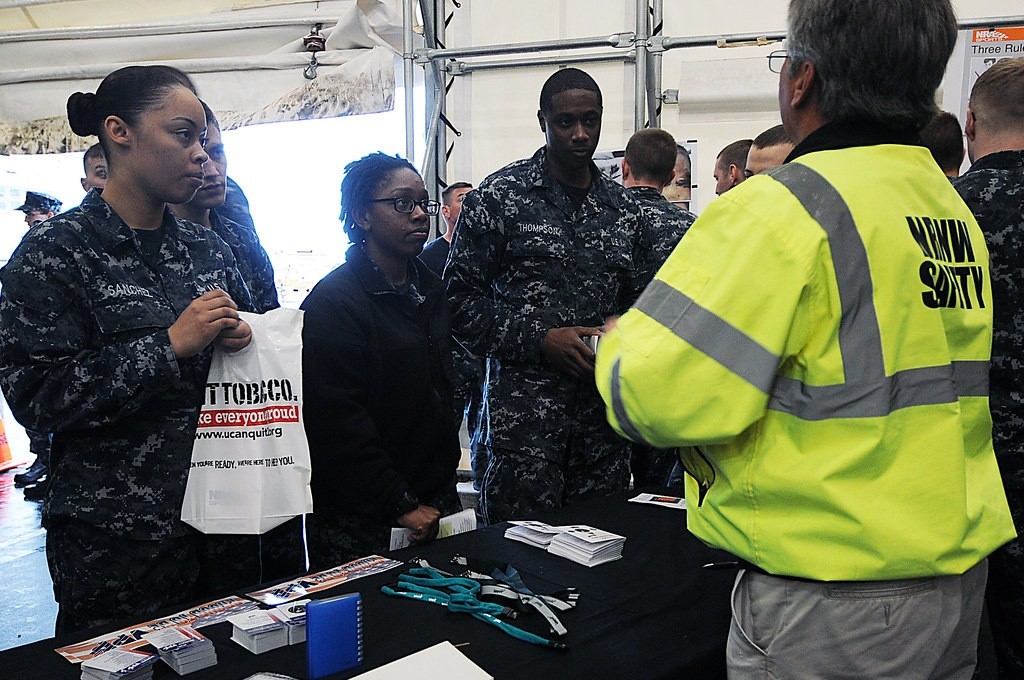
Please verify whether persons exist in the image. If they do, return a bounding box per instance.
[440,67,661,530]
[423,121,801,508]
[299,151,446,571]
[983,57,997,69]
[12,96,276,518]
[580,0,1016,680]
[932,58,1024,680]
[0,63,304,637]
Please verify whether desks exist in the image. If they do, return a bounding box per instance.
[0,487,748,680]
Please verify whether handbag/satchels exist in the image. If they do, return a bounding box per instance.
[181,307,313,534]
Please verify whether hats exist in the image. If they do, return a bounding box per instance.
[14,191,62,213]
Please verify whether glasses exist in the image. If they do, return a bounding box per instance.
[369,197,441,216]
[767,50,800,74]
